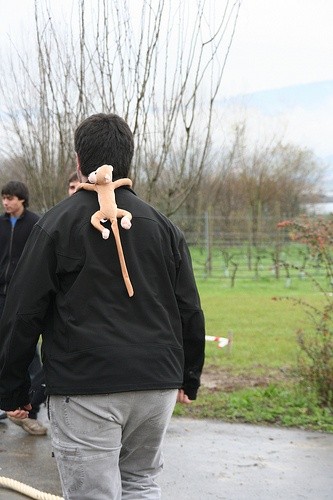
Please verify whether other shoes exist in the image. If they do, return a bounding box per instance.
[8,414,47,435]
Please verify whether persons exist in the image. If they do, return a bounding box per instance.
[0,115,206,500]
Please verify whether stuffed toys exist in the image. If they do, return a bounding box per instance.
[74,164,134,297]
